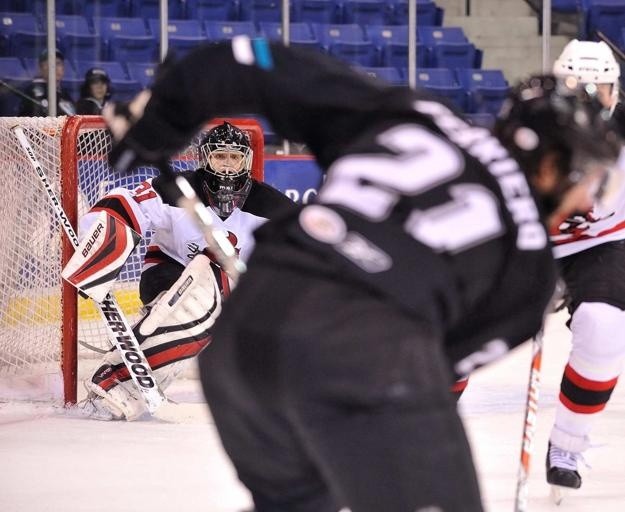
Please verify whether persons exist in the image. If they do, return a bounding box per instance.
[109,36,623,512]
[76,66,113,115]
[62,121,299,422]
[19,47,76,115]
[452,38,625,490]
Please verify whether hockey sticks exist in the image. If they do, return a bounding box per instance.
[514,330,564,510]
[10,124,216,423]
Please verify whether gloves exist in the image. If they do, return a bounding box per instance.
[105,81,196,174]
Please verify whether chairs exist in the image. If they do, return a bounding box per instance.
[2,1,514,144]
[529,1,624,71]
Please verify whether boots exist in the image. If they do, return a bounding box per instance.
[544,421,590,492]
[81,347,128,421]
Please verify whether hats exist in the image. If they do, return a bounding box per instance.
[85,67,111,85]
[40,46,64,63]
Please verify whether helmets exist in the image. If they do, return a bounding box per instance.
[491,74,623,164]
[198,121,255,158]
[552,37,621,121]
